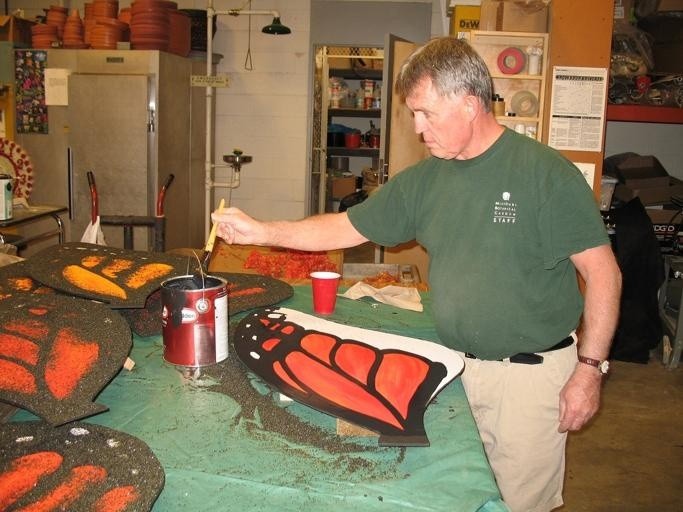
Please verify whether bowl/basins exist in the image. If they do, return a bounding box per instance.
[31,0,192,56]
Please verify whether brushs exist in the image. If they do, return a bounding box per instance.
[191,198,225,289]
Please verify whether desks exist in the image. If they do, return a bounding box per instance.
[1,261,511,512]
[1,204,69,258]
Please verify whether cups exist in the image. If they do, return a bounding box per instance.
[309,272,341,315]
[327,131,360,149]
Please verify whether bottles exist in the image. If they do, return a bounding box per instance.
[0,174,13,220]
[492,98,505,116]
[369,134,379,148]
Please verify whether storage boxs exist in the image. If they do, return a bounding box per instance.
[601,152,683,258]
[446,0,549,41]
[326,177,355,201]
[613,0,634,27]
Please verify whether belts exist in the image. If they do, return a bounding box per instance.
[464,333,573,365]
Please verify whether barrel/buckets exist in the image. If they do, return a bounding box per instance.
[159,248,230,366]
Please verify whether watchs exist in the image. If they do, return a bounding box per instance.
[578,356,608,375]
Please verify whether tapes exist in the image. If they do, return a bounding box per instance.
[510,90,538,118]
[497,47,526,75]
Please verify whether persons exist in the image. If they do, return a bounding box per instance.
[210,35,625,512]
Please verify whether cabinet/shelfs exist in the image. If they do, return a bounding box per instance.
[311,43,388,213]
[470,30,549,141]
